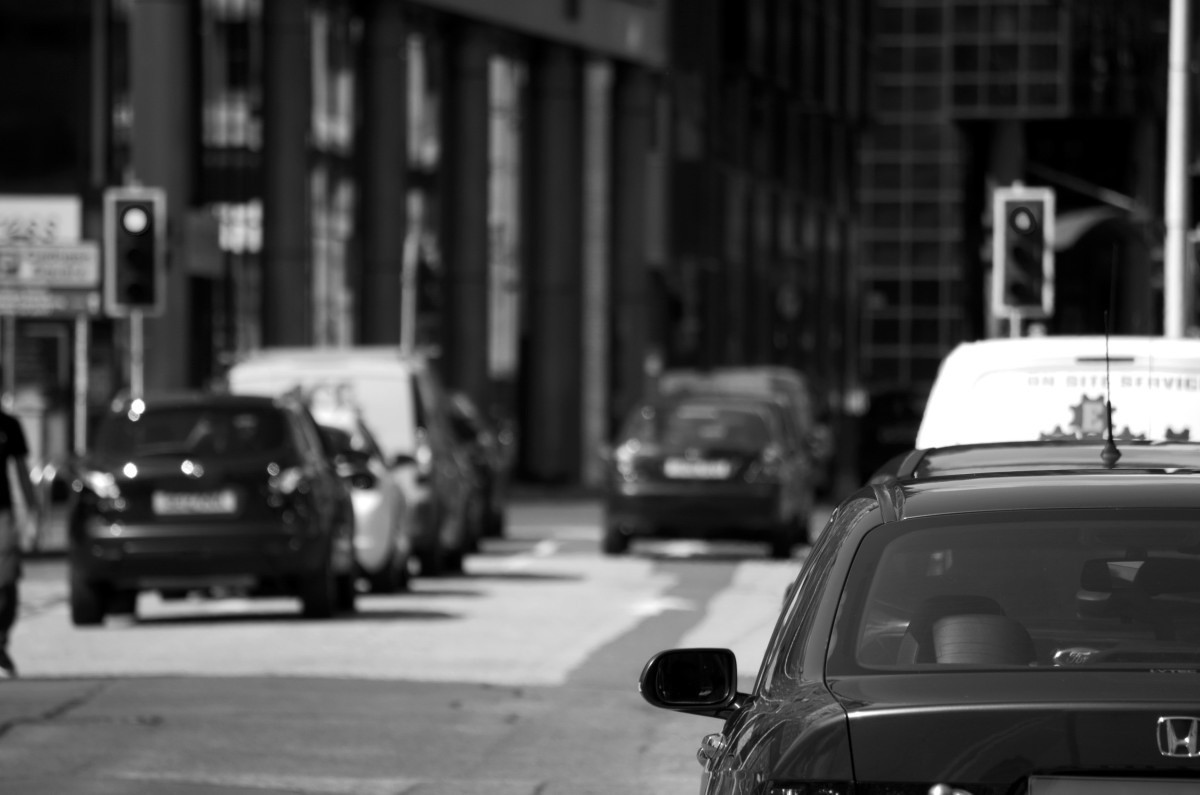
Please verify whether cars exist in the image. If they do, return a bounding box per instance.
[636,461,1200,795]
[854,438,1200,492]
[37,385,369,628]
[593,361,839,562]
[223,341,518,598]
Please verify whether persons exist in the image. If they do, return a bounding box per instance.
[0,408,41,671]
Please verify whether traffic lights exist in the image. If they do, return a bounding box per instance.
[101,185,168,318]
[991,184,1055,321]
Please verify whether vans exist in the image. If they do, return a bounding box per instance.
[911,330,1200,459]
